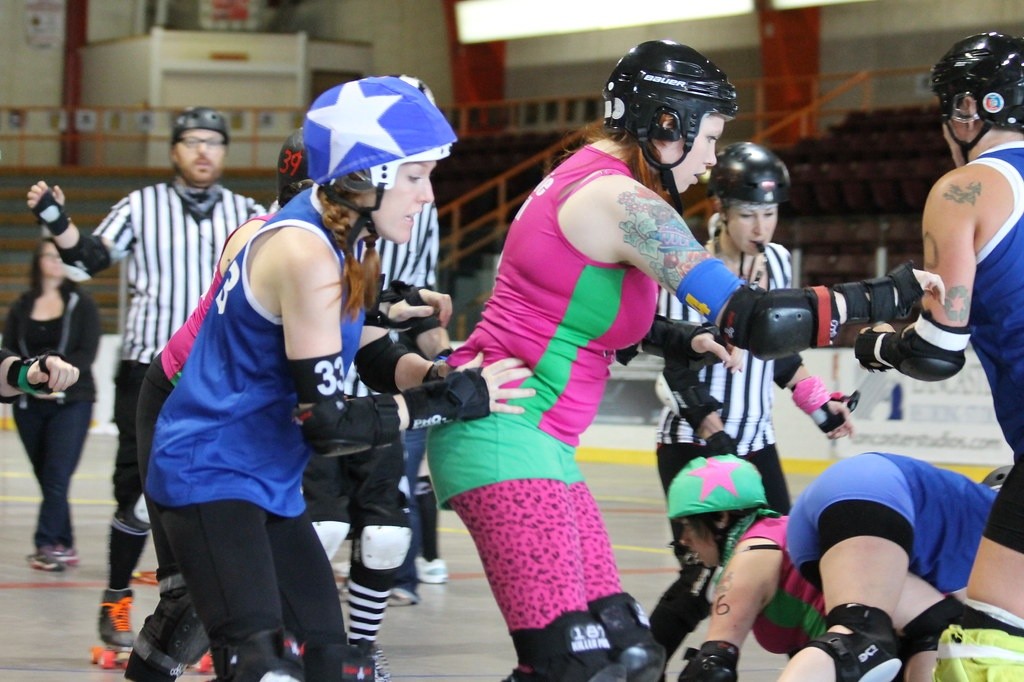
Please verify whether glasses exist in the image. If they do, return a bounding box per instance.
[179,136,225,148]
[40,252,61,261]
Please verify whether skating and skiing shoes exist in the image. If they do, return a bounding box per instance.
[91,588,136,669]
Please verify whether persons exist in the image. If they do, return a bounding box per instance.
[856,31,1024,636]
[28,106,266,671]
[123,75,535,682]
[648,143,861,682]
[667,453,1024,682]
[425,38,946,682]
[0,348,80,405]
[3,237,100,571]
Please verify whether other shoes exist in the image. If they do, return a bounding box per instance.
[415,555,448,583]
[387,588,419,605]
[26,551,68,571]
[51,545,79,566]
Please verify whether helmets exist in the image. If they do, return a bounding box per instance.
[602,40,740,143]
[172,106,228,145]
[707,143,790,204]
[303,76,457,185]
[666,454,768,519]
[276,128,315,207]
[389,75,436,107]
[930,33,1024,131]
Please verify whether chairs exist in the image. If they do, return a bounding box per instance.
[774,100,966,285]
[431,126,583,249]
